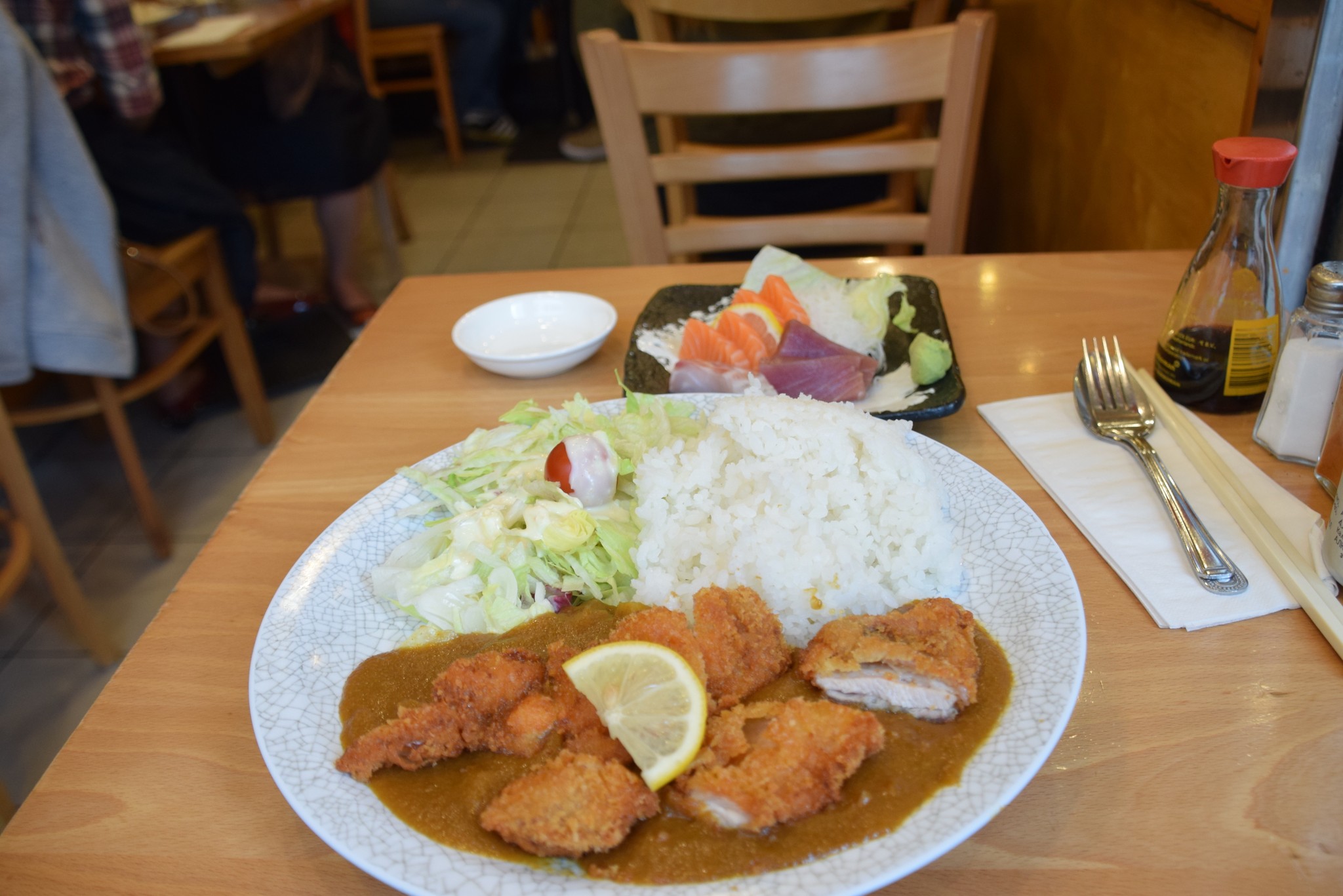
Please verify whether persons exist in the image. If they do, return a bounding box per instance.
[0,0,255,427]
[560,0,630,158]
[171,13,382,326]
[676,10,901,259]
[374,0,526,143]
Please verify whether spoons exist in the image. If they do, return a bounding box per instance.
[1072,351,1249,594]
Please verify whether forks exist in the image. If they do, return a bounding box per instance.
[1081,335,1236,580]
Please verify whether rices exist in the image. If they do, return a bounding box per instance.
[627,370,961,650]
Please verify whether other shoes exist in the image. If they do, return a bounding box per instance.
[332,300,378,347]
[152,348,209,426]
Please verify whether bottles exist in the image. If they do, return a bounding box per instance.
[1250,259,1343,588]
[1151,136,1298,415]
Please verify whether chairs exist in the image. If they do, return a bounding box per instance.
[0,401,118,671]
[619,1,947,257]
[3,230,278,559]
[577,10,998,259]
[352,0,465,160]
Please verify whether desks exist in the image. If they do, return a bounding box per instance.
[142,1,342,425]
[0,246,1343,896]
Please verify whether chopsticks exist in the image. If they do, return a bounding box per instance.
[1121,356,1343,660]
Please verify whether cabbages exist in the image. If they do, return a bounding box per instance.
[368,365,708,634]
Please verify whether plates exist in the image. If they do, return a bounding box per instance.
[451,290,618,379]
[621,273,967,420]
[246,391,1086,896]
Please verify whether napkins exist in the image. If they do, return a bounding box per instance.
[978,390,1339,633]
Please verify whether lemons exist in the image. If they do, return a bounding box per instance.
[559,641,706,792]
[716,302,782,360]
